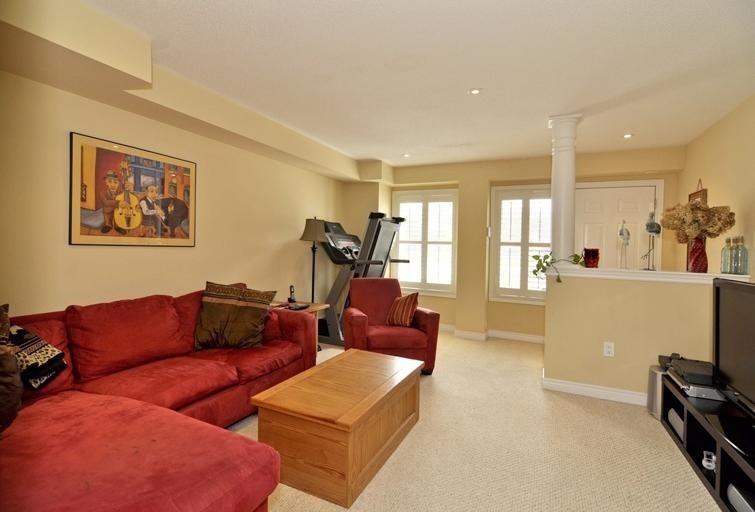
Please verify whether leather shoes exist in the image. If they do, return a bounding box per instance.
[101,225,127,233]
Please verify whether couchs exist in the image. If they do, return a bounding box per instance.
[342,277,440,376]
[0,283,319,512]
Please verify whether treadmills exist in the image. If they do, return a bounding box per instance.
[318,212,409,347]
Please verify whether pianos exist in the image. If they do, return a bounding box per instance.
[153,198,188,237]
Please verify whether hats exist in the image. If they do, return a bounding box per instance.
[103,169,119,180]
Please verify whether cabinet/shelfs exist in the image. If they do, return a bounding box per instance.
[661,373,755,512]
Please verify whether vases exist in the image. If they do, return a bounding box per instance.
[688,237,708,273]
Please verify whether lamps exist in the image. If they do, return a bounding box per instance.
[299,217,329,352]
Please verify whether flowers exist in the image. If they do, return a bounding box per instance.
[660,197,736,244]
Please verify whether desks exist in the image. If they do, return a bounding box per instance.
[276,301,330,320]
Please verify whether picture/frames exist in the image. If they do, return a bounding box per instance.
[69,132,196,248]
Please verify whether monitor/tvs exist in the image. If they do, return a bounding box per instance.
[712,277,755,420]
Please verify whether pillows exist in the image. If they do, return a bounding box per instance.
[194,281,277,349]
[1,303,23,431]
[385,292,419,328]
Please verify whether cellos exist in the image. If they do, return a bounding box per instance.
[113,160,142,231]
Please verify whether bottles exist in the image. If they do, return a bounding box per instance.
[720,238,731,273]
[730,238,738,273]
[734,235,748,275]
